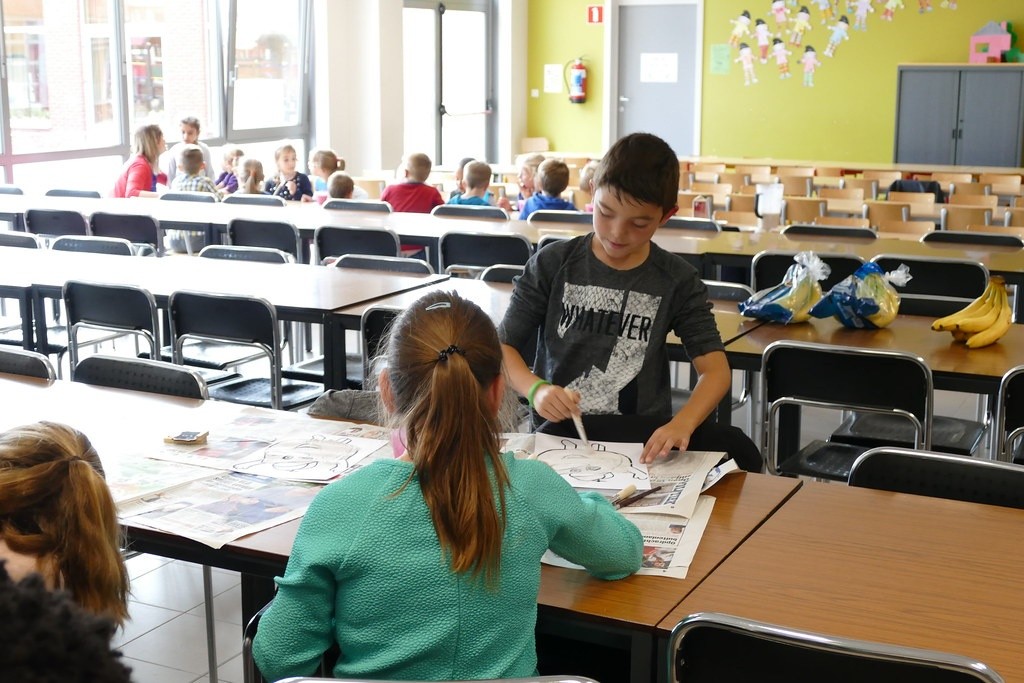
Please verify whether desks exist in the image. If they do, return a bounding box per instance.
[329,275,769,454]
[0,245,55,352]
[655,483,1024,683]
[32,251,450,391]
[722,310,1024,474]
[0,373,803,683]
[0,194,719,269]
[703,232,1024,287]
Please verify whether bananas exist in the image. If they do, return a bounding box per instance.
[830,267,900,327]
[931,275,1011,348]
[769,270,822,323]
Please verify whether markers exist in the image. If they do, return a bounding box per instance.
[611,484,635,506]
[563,386,588,446]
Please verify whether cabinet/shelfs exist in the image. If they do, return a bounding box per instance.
[891,64,1024,169]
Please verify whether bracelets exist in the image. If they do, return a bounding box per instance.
[529,379,551,409]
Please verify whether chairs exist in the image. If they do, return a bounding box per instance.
[0,163,1024,683]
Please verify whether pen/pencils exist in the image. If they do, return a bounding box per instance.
[615,486,662,510]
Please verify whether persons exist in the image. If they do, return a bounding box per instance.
[0,421,135,683]
[250,291,643,681]
[114,116,599,279]
[492,132,732,463]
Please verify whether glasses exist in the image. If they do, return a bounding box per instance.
[366,355,391,380]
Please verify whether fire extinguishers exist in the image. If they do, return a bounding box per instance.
[564,54,589,103]
